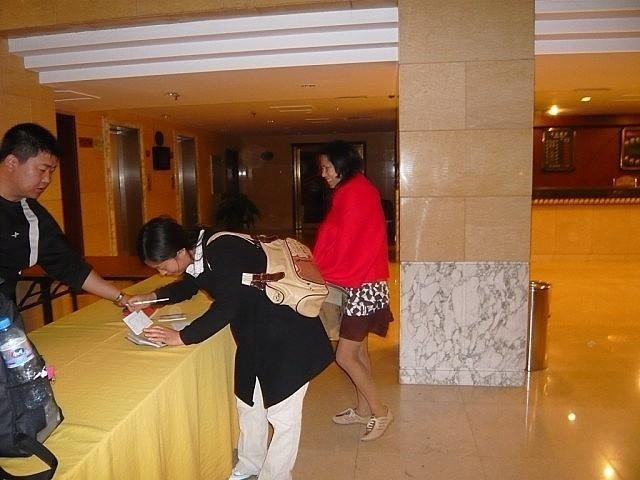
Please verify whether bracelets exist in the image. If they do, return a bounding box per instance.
[112,291,124,305]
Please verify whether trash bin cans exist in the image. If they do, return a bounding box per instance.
[526,281,552,370]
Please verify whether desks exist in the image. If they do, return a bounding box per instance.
[0,255,231,480]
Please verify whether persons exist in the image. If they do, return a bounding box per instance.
[125,215,336,480]
[1,122,142,334]
[308,137,395,444]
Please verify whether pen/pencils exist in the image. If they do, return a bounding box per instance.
[125,297,170,305]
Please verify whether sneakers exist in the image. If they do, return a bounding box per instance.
[332,407,373,427]
[360,404,395,443]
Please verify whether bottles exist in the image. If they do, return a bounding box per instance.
[0,317,52,409]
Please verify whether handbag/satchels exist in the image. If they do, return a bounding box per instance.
[0,277,66,480]
[201,230,330,319]
